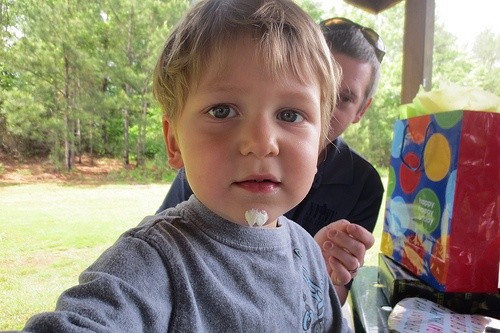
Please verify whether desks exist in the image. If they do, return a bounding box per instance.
[348,265,392,333]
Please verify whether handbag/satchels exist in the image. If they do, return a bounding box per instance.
[380,109,500,293]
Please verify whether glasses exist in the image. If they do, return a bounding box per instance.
[320,16,386,63]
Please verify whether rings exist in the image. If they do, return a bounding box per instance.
[348,261,360,273]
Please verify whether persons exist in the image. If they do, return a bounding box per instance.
[1,0,354,333]
[156,16,385,306]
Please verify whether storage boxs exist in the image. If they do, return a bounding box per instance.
[377,252,500,319]
